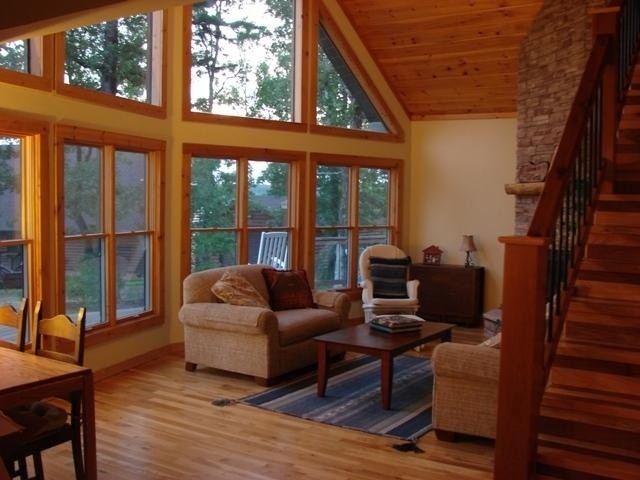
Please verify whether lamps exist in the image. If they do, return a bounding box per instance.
[457,234,481,266]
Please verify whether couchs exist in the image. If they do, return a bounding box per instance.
[358,241,424,321]
[180,260,349,384]
[428,331,502,447]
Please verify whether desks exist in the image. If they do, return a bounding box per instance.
[0,346,97,480]
[310,314,457,408]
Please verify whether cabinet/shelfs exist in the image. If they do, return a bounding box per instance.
[407,264,488,328]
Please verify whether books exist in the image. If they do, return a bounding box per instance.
[369,322,422,333]
[371,316,423,329]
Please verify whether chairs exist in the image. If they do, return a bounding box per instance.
[0,298,30,352]
[257,230,288,270]
[0,300,88,480]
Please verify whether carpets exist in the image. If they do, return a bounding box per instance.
[238,347,436,445]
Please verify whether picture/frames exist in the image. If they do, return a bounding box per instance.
[422,244,443,265]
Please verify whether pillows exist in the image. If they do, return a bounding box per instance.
[261,265,317,312]
[367,253,410,298]
[208,272,270,314]
